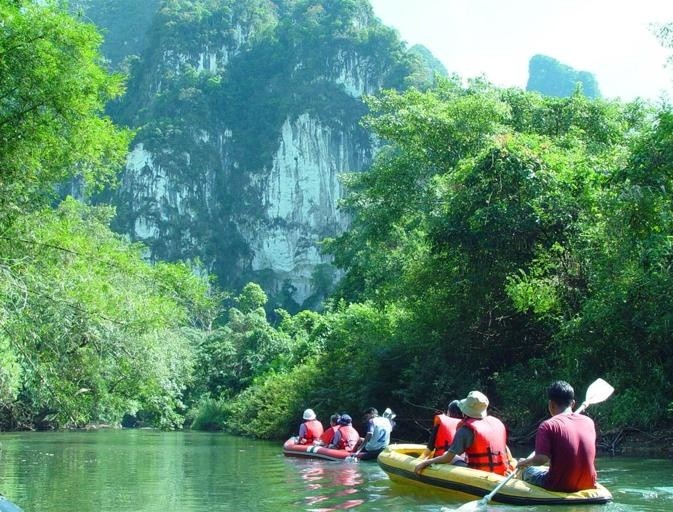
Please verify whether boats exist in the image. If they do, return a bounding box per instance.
[282,434,381,462]
[376,442,613,504]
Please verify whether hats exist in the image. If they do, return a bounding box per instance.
[303,409,316,420]
[458,391,489,418]
[337,414,352,426]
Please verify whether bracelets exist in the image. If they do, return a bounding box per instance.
[422,460,429,466]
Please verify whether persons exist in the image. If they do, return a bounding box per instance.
[319,413,341,448]
[414,390,508,476]
[517,380,597,493]
[424,399,465,458]
[352,406,392,453]
[297,408,324,445]
[328,414,362,452]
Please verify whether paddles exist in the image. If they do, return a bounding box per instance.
[344,407,391,461]
[457,378,614,511]
